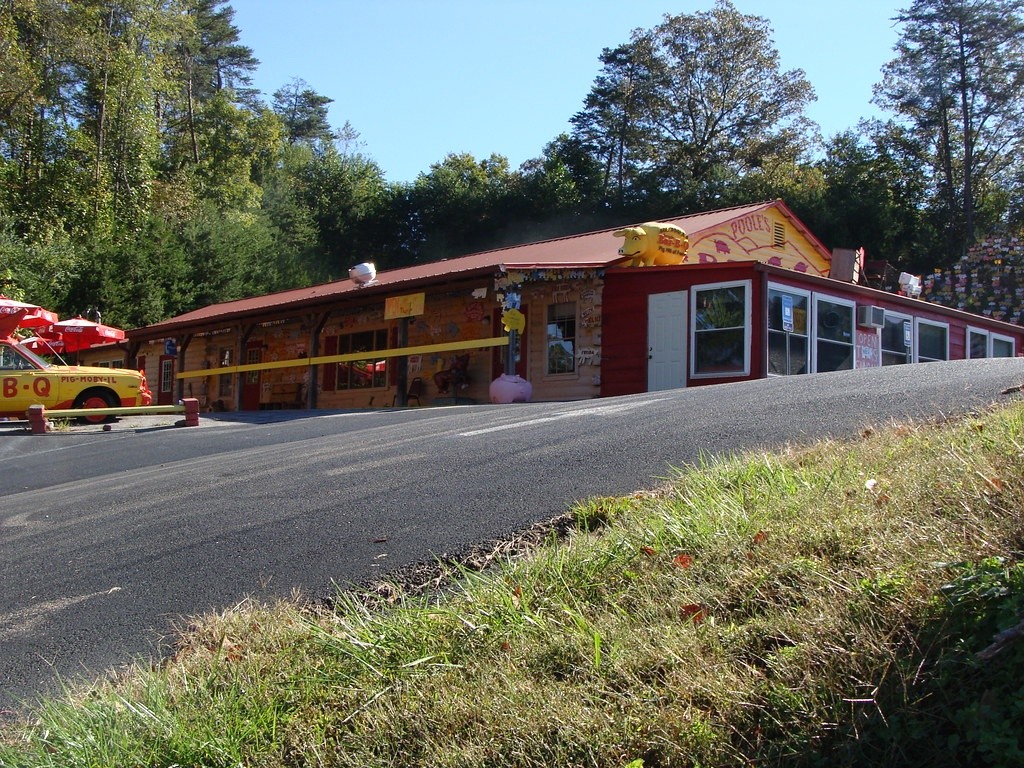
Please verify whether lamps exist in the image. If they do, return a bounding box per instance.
[260,344,268,351]
[481,315,491,326]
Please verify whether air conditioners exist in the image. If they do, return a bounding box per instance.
[858,305,885,328]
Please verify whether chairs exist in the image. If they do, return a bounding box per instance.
[392,377,423,407]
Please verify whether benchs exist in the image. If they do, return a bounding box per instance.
[257,382,305,409]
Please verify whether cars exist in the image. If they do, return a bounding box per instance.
[0,339,151,425]
